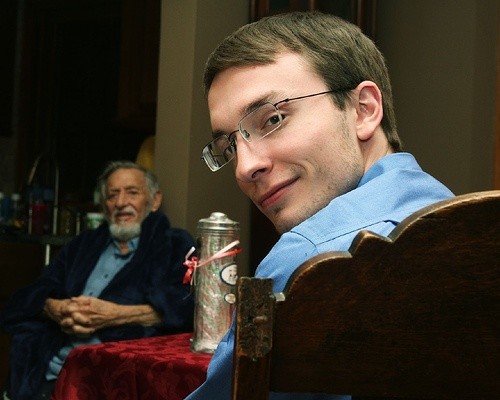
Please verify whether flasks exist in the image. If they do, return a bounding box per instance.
[190,212,241,355]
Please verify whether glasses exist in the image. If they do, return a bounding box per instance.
[199,88,355,173]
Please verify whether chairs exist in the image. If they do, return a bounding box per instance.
[233,189,500,399]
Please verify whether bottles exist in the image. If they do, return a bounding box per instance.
[85,188,105,232]
[0,192,76,235]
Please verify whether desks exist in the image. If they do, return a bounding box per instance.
[52,332,213,400]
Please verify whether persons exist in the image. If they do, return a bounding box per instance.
[185,11,458,399]
[0,160,199,399]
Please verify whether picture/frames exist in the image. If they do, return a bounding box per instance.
[257,0,376,46]
